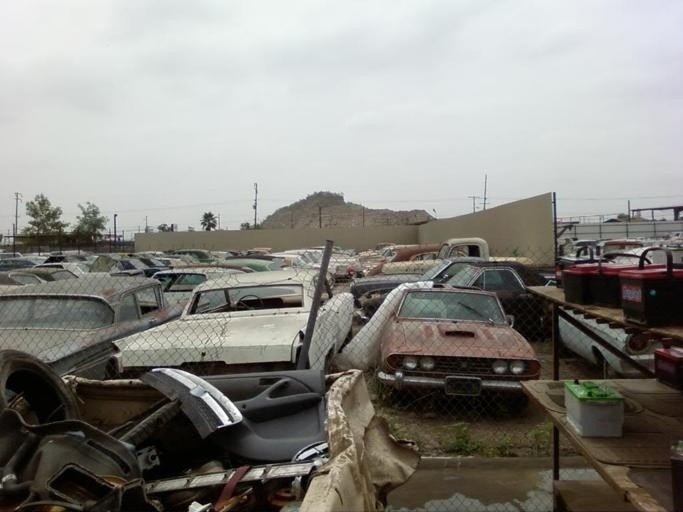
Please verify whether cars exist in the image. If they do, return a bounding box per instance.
[571,233,683,260]
[559,303,673,378]
[0,348,420,512]
